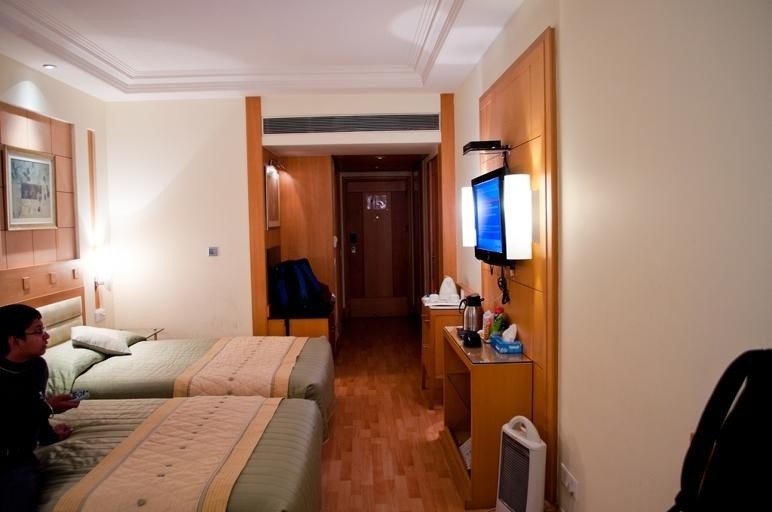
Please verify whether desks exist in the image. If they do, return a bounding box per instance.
[125,327,164,341]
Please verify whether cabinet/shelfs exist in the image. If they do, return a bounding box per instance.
[421,304,464,410]
[438,326,532,511]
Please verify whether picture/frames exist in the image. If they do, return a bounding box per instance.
[2,144,59,232]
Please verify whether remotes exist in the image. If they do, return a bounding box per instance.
[70,388,89,402]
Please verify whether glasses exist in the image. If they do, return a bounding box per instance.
[23,325,48,337]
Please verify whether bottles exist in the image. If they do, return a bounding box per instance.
[493,307,506,336]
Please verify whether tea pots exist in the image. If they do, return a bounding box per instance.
[458,294,485,333]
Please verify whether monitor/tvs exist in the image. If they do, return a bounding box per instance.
[471,167,506,265]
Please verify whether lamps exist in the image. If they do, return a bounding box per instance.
[94,278,112,309]
[269,159,287,171]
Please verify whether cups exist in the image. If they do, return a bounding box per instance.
[462,331,482,349]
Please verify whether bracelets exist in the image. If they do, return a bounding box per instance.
[45,398,55,419]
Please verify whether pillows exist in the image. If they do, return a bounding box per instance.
[71,325,146,356]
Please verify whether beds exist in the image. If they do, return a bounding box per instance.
[36,296,335,446]
[33,395,321,512]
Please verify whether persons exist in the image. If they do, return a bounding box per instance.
[1,304,80,512]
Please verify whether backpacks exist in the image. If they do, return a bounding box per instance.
[663,344,770,512]
[267,254,335,318]
